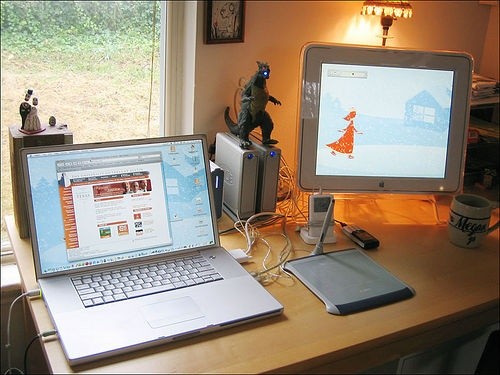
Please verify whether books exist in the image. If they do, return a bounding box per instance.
[472,72,498,100]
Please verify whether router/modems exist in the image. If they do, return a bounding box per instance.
[282,198,417,318]
[208,159,226,220]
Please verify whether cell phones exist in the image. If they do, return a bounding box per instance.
[341,222,381,252]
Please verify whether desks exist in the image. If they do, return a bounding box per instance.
[3,159,499,375]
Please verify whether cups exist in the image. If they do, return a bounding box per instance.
[448,194,500,248]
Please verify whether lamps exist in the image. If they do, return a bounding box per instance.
[357,1,415,52]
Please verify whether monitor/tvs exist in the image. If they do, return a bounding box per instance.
[294,39,475,199]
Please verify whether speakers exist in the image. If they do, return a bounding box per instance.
[214,133,261,226]
[249,130,282,221]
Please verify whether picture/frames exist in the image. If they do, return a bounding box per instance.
[204,3,248,47]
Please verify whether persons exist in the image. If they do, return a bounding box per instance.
[131,182,143,193]
[122,182,133,194]
[19,88,34,129]
[24,97,40,131]
[141,180,149,192]
[218,2,239,34]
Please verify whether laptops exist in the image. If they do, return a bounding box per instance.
[17,132,285,369]
